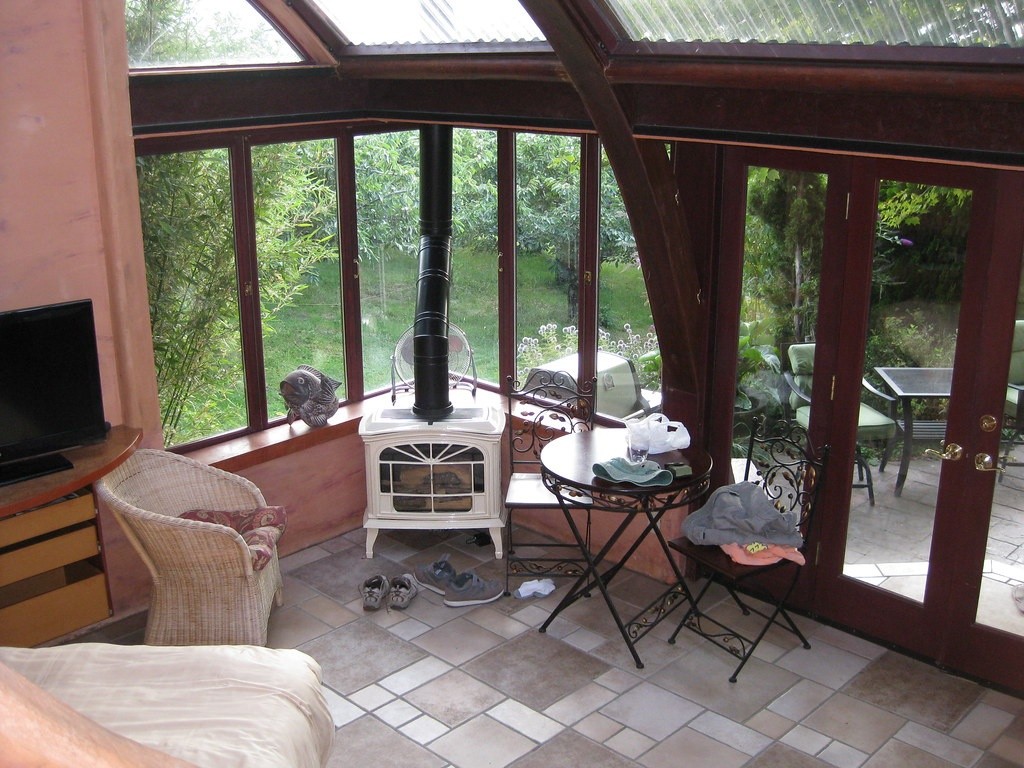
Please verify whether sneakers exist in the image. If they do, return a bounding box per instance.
[387,574,417,610]
[358,571,390,612]
[442,568,505,607]
[412,558,456,596]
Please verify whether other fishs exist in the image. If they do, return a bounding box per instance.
[278,364,342,428]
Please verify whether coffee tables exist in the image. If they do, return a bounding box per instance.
[536,427,701,669]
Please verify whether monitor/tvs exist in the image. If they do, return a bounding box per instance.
[0,299,108,467]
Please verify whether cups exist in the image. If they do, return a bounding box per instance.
[629,434,649,464]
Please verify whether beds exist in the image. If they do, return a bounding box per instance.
[0,642,337,768]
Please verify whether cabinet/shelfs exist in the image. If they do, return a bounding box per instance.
[0,424,144,648]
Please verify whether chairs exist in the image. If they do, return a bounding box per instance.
[504,369,597,597]
[668,417,832,685]
[995,319,1024,483]
[93,447,289,647]
[780,342,897,509]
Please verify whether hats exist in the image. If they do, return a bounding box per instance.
[591,457,675,489]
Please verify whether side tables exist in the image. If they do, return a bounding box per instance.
[357,404,507,560]
[873,366,954,499]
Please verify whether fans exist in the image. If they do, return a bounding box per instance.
[389,320,478,407]
